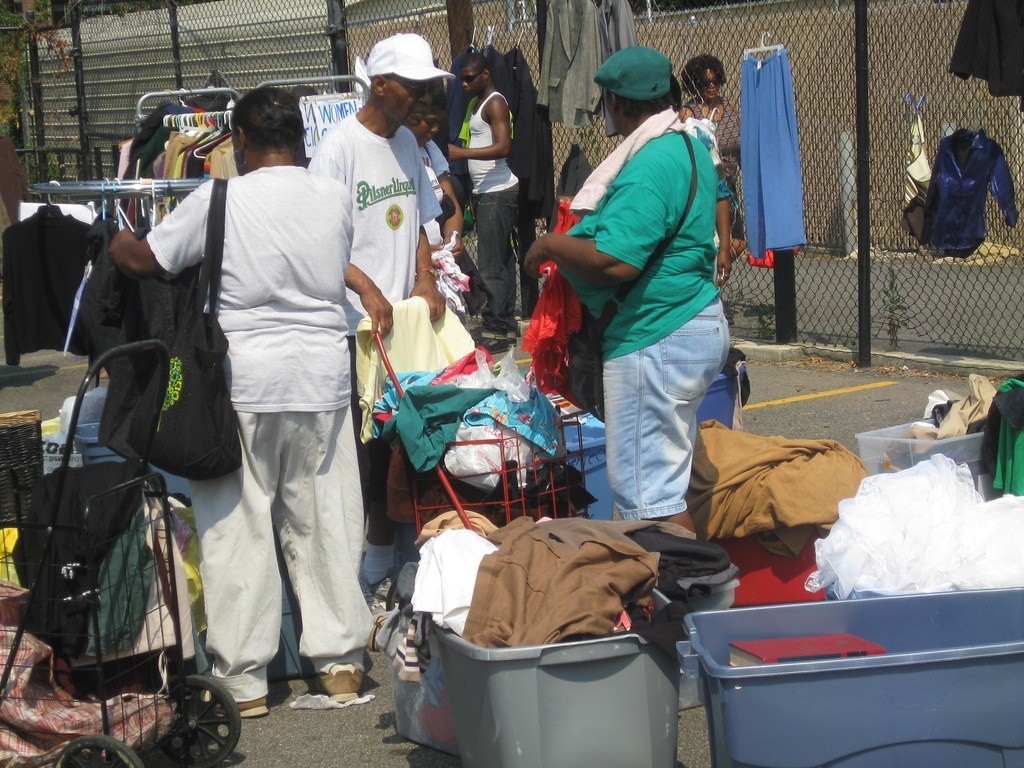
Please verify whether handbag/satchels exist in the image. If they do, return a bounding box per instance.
[97,177,243,479]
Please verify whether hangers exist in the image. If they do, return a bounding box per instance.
[905,92,927,113]
[282,78,368,102]
[433,27,524,60]
[743,32,783,61]
[161,85,235,110]
[163,110,233,160]
[35,177,176,230]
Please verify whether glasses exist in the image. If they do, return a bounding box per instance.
[700,79,718,86]
[461,71,480,79]
[385,79,443,98]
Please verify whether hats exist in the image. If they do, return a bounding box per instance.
[366,32,458,82]
[593,47,670,100]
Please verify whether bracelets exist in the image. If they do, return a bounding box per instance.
[416,265,439,278]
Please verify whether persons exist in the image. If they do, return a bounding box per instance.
[524,43,746,534]
[307,32,520,585]
[109,87,377,718]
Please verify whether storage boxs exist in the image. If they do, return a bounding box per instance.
[855,419,1002,502]
[677,587,1024,768]
[433,624,677,768]
[74,423,191,499]
[563,425,614,521]
[169,573,315,694]
[677,581,741,710]
[714,527,826,607]
[393,658,460,757]
[695,365,746,431]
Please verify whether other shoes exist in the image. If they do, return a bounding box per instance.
[470,327,518,354]
[213,695,268,718]
[315,663,365,703]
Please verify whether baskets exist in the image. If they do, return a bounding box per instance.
[0,409,45,521]
